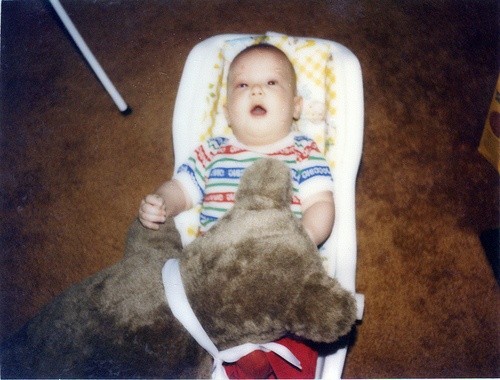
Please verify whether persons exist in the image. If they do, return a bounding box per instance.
[137,43,334,380]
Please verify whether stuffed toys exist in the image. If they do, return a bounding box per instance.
[0,159,357,380]
